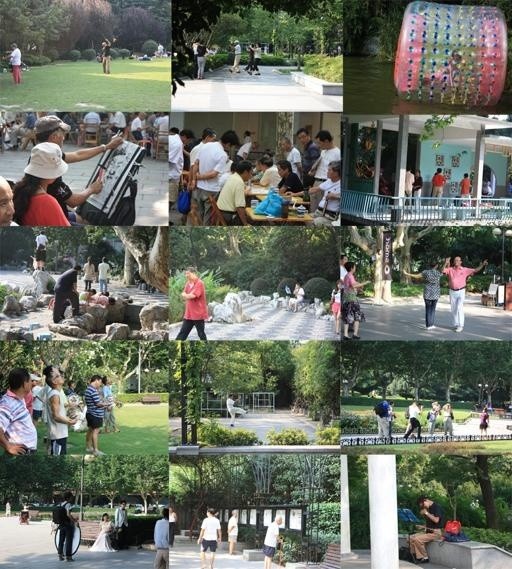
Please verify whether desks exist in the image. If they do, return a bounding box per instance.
[177,169,314,226]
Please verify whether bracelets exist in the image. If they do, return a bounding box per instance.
[425,511,428,515]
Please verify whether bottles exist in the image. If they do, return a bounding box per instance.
[303,188,310,202]
[286,186,292,199]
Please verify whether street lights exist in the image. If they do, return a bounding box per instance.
[493,225,511,283]
[478,383,488,399]
[70,455,95,521]
[144,367,160,393]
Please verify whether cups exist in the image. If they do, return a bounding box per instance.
[296,204,309,218]
[251,199,259,209]
[281,200,289,218]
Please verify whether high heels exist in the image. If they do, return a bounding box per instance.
[353,334,360,339]
[343,336,352,340]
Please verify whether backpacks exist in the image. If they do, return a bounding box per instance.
[52,502,69,524]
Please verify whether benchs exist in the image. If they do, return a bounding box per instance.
[295,544,340,568]
[399,534,512,569]
[77,521,100,548]
[142,396,161,404]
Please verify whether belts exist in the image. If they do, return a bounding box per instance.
[451,287,466,291]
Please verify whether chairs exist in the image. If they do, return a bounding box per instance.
[0,113,169,162]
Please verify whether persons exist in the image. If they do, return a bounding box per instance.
[193,37,200,78]
[414,169,423,206]
[28,229,117,328]
[12,142,72,226]
[441,256,489,332]
[285,283,305,312]
[26,115,122,226]
[0,113,168,158]
[88,513,117,552]
[226,395,249,426]
[229,40,242,74]
[340,262,371,340]
[99,39,111,74]
[0,366,120,455]
[330,279,340,334]
[460,173,470,198]
[432,49,466,91]
[175,267,208,341]
[153,508,168,569]
[169,127,341,226]
[405,165,415,206]
[169,506,177,547]
[197,508,222,569]
[248,42,261,75]
[407,495,444,562]
[432,168,445,197]
[403,257,452,329]
[263,516,284,569]
[227,510,239,555]
[0,175,20,226]
[52,492,79,561]
[379,167,391,197]
[197,39,206,79]
[115,500,129,528]
[341,255,349,289]
[374,400,493,441]
[0,42,21,84]
[243,44,254,71]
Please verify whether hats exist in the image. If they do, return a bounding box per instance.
[33,115,71,134]
[24,142,68,179]
[30,374,41,380]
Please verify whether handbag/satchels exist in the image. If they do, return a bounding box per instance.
[445,521,461,535]
[38,244,45,249]
[178,192,190,213]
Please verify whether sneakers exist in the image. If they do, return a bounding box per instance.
[86,448,94,451]
[456,327,463,332]
[424,325,435,330]
[93,451,104,456]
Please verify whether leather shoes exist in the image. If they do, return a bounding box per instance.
[412,558,429,564]
